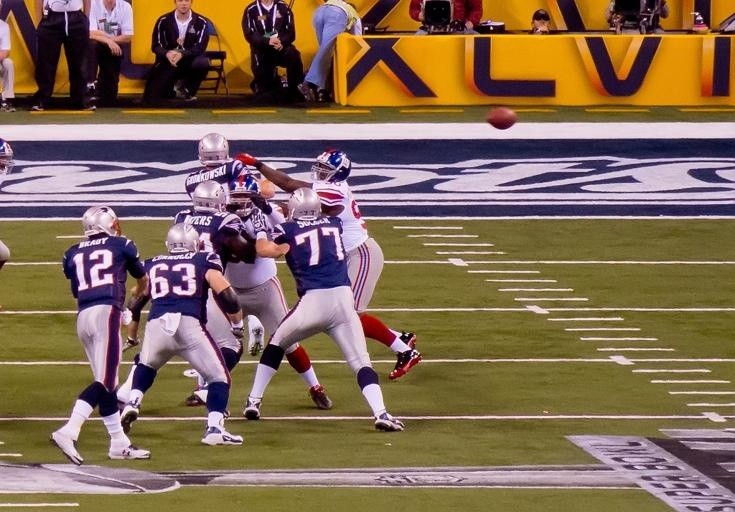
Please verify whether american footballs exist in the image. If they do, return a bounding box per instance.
[488,107,517,129]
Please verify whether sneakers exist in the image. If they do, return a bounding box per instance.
[195,387,210,402]
[183,368,198,377]
[184,94,198,101]
[374,411,404,430]
[50,429,83,465]
[186,389,204,406]
[310,387,332,410]
[317,92,329,101]
[80,101,95,111]
[109,443,151,460]
[400,331,417,351]
[248,327,264,357]
[244,398,261,418]
[33,105,44,110]
[1,102,16,113]
[298,83,312,101]
[390,349,421,380]
[120,404,139,433]
[200,424,243,445]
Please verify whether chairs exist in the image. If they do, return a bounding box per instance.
[177,15,229,94]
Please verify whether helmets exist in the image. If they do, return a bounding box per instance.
[230,178,260,213]
[197,135,228,162]
[0,138,14,171]
[194,181,224,213]
[289,189,322,218]
[81,207,122,238]
[166,223,199,253]
[312,150,352,182]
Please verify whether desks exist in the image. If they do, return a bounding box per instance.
[333,29,735,108]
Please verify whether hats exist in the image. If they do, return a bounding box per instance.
[532,9,549,19]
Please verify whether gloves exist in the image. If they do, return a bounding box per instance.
[230,322,245,338]
[252,195,272,215]
[122,336,138,351]
[250,212,269,232]
[235,153,262,170]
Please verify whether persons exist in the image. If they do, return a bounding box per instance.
[185,133,265,359]
[46,204,150,467]
[173,180,256,419]
[297,0,363,102]
[86,0,134,105]
[185,179,334,410]
[606,0,670,29]
[530,9,551,34]
[236,147,422,382]
[31,0,96,111]
[408,0,483,30]
[0,1,16,113]
[244,187,405,434]
[120,224,245,444]
[144,0,211,102]
[241,0,304,103]
[0,138,14,270]
